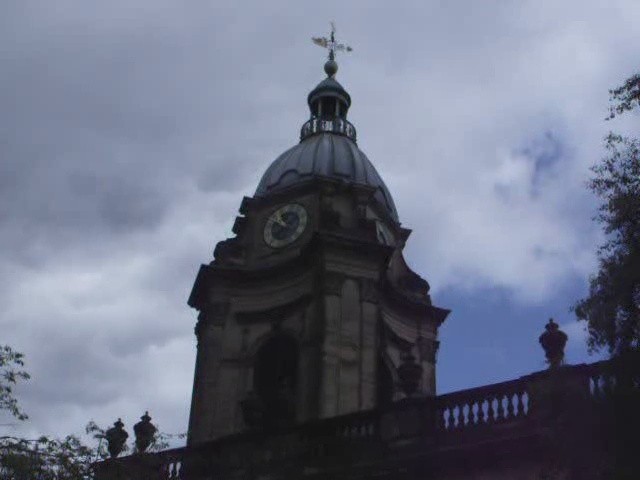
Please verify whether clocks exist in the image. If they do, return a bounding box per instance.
[262,202,308,250]
[373,217,395,270]
